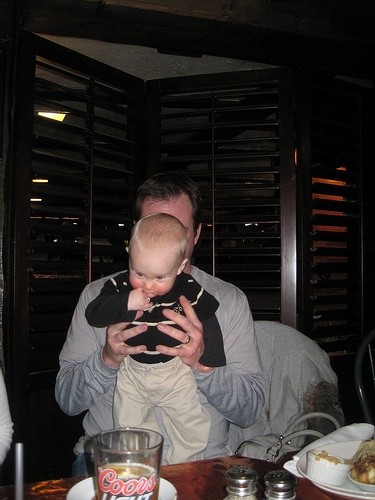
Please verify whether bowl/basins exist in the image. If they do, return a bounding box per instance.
[306,451,352,486]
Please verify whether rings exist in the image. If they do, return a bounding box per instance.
[148,297,150,301]
[182,334,190,344]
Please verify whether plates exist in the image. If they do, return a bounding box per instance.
[67,476,178,500]
[311,481,375,500]
[296,440,375,497]
[346,469,375,493]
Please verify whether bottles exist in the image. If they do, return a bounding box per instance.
[263,470,297,500]
[223,467,259,500]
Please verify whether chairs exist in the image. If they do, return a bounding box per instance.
[356,330,375,426]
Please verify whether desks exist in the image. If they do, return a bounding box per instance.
[0,455,336,500]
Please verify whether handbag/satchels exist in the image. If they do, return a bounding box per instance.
[235,411,341,464]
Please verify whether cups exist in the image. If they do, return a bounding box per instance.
[89,427,164,500]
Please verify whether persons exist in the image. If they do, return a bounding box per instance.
[0,368,14,464]
[84,212,227,464]
[55,168,265,477]
